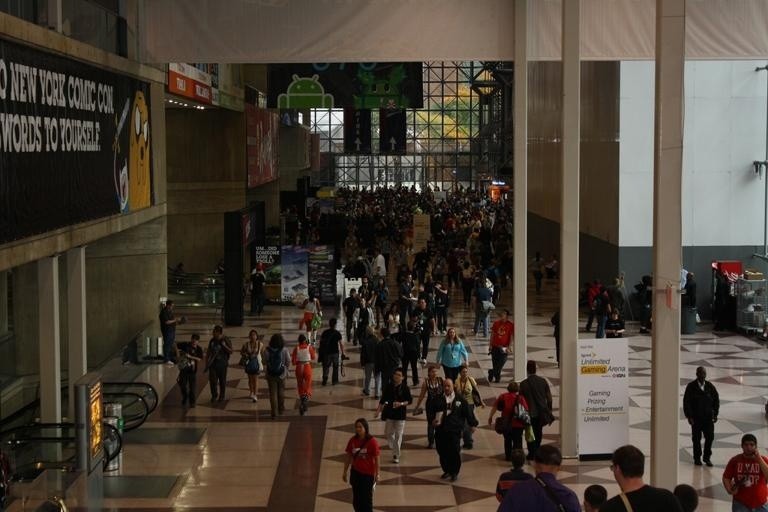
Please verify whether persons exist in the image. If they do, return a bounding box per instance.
[722,432,768,511]
[374,327,484,481]
[177,317,422,415]
[250,261,266,317]
[159,300,181,364]
[545,254,558,280]
[495,446,699,510]
[342,417,380,511]
[683,365,721,466]
[640,268,696,334]
[488,360,556,460]
[551,310,561,368]
[488,309,513,383]
[585,278,625,338]
[281,184,509,343]
[528,251,545,295]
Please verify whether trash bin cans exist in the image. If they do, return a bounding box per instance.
[103,404,122,471]
[681,305,698,334]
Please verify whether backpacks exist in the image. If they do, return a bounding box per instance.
[266,346,284,375]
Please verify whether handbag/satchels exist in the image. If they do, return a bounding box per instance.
[513,403,531,426]
[472,388,482,407]
[240,358,249,366]
[311,316,322,329]
[481,300,496,312]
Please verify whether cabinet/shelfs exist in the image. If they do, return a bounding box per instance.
[737,279,768,329]
